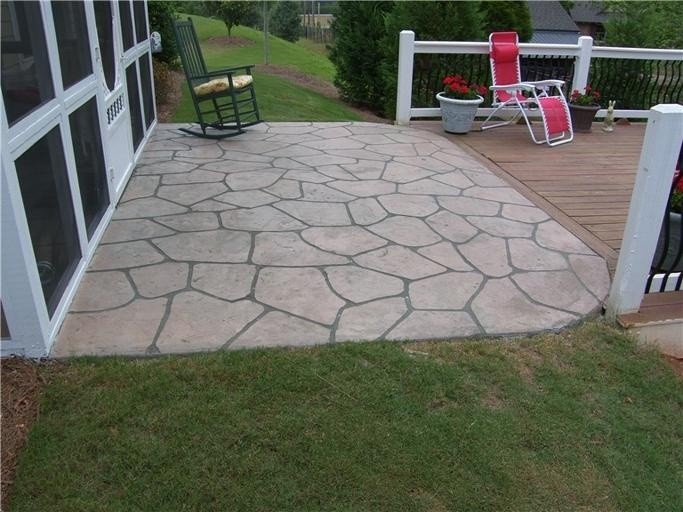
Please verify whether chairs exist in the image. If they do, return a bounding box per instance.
[166,15,267,140]
[483,31,577,148]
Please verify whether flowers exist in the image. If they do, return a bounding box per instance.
[561,78,598,108]
[438,67,489,102]
[665,164,683,213]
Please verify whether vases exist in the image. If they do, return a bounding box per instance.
[650,210,683,272]
[561,100,598,134]
[434,91,482,133]
